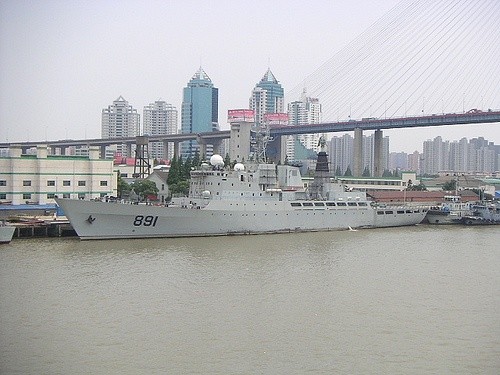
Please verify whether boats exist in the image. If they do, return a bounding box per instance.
[360,207,429,229]
[426,204,499,225]
[0,227,16,244]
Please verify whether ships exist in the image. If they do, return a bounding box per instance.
[55,170,375,241]
